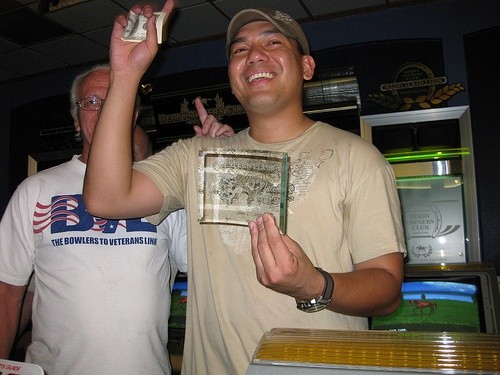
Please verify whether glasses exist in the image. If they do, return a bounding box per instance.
[77,96,106,111]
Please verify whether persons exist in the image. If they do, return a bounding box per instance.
[83,0,408,375]
[1,62,235,375]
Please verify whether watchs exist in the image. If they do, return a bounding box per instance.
[293,268,335,313]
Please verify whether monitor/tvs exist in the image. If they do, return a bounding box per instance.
[369,273,494,334]
[166,277,190,336]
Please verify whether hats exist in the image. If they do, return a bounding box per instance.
[225,8,312,57]
